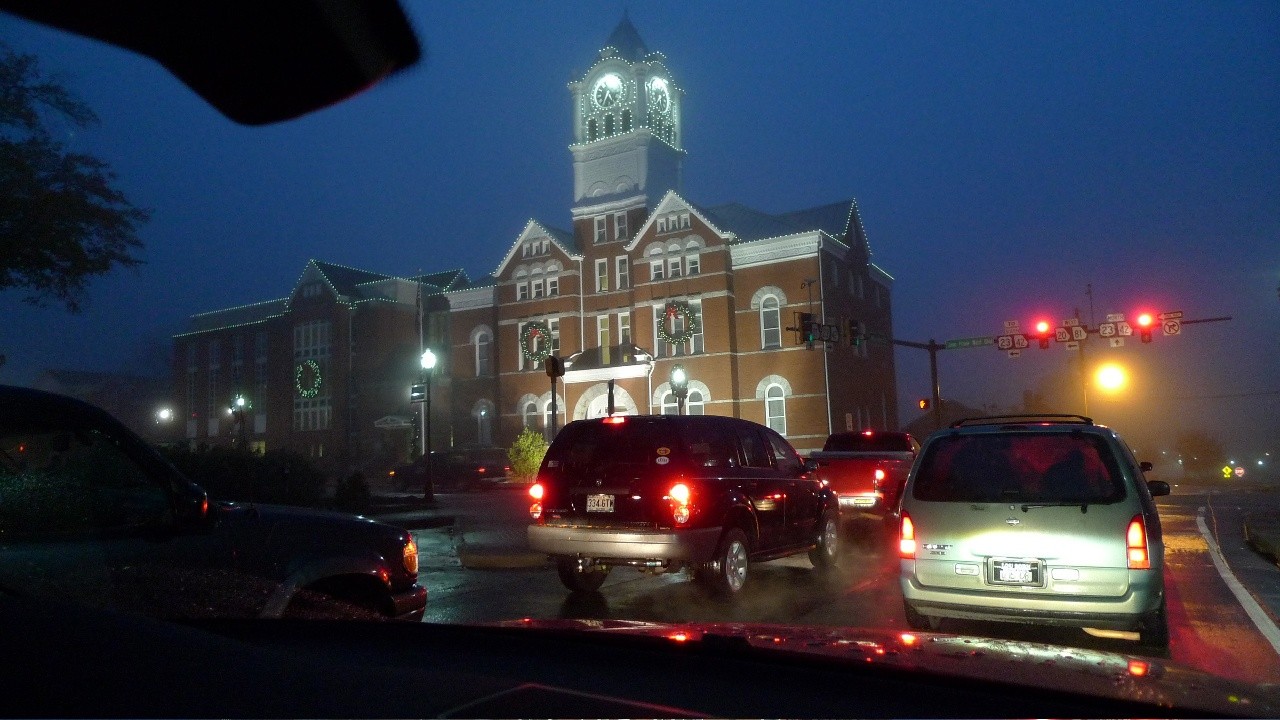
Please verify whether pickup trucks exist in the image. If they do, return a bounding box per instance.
[806,428,921,533]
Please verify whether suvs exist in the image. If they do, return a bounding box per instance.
[386,444,514,493]
[897,411,1169,653]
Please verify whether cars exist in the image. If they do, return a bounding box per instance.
[0,383,428,639]
[526,410,842,599]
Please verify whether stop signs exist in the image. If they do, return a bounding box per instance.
[1235,466,1246,478]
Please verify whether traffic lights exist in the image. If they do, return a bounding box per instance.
[1036,319,1049,349]
[798,312,814,344]
[848,320,860,346]
[919,399,930,410]
[1136,313,1152,343]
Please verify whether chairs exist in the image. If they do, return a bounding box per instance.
[1039,464,1093,505]
[931,464,977,505]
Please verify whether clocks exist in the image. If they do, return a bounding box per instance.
[594,77,621,107]
[652,79,668,113]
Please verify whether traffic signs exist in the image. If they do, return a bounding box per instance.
[1157,311,1183,320]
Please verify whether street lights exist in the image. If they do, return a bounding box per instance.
[418,347,439,503]
